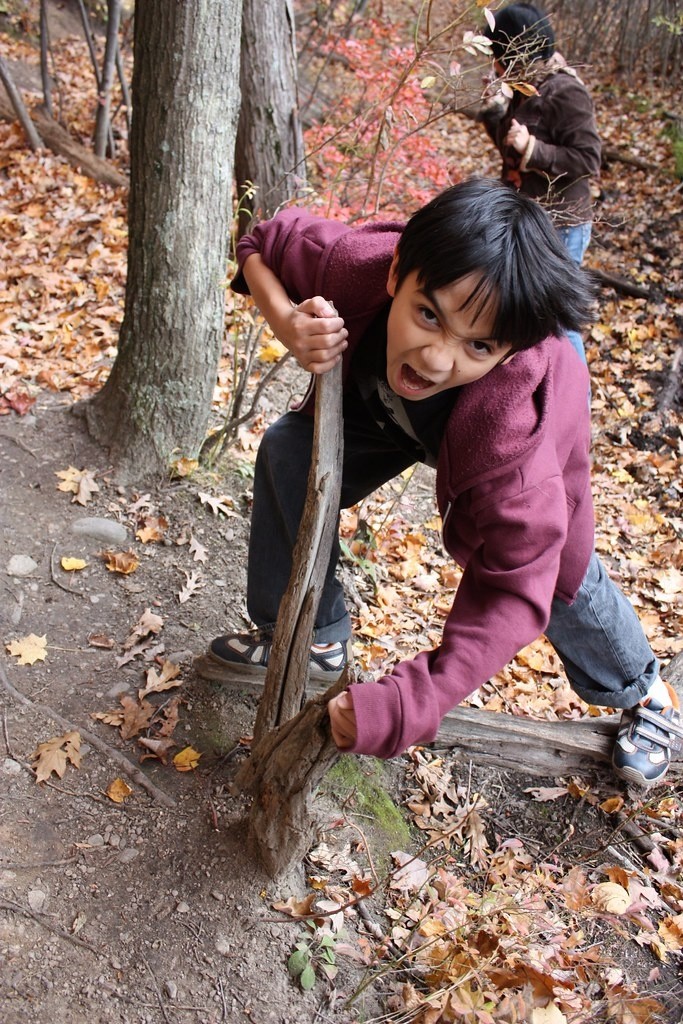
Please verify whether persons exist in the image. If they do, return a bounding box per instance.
[208,177,680,787]
[483,0,601,414]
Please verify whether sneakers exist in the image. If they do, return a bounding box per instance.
[209,622,348,681]
[611,677,683,785]
